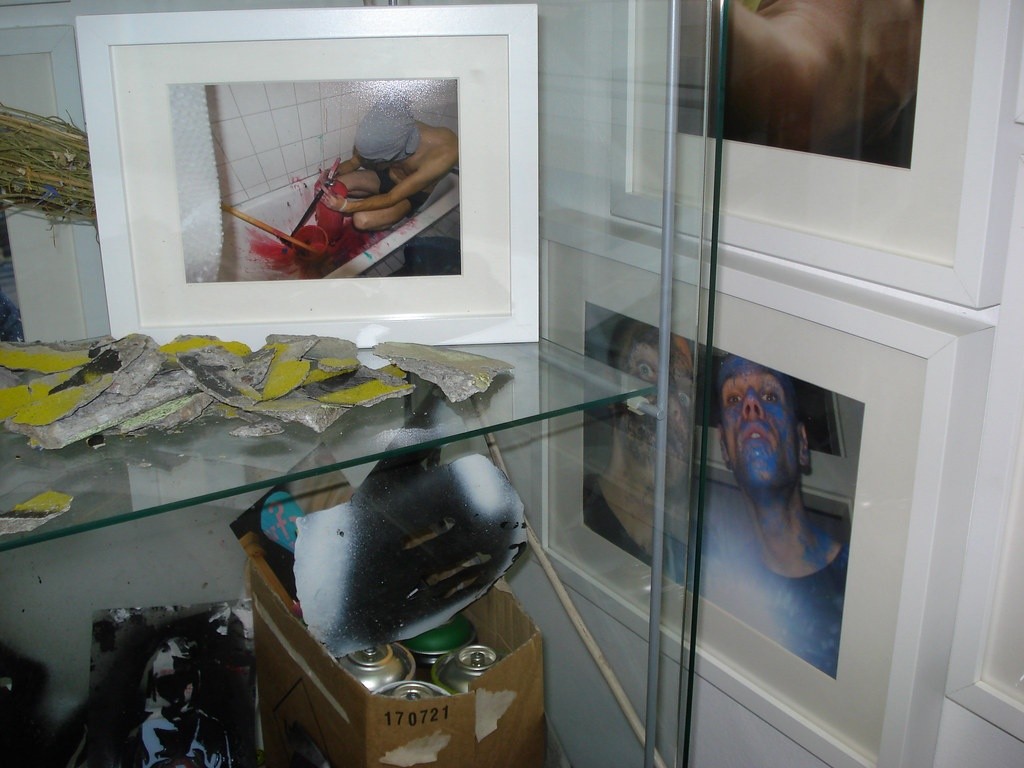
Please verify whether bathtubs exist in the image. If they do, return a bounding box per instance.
[220,156,460,279]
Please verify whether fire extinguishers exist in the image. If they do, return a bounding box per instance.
[313,157,349,245]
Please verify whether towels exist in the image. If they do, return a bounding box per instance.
[169,84,226,282]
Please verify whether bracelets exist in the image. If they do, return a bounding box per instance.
[339,199,347,211]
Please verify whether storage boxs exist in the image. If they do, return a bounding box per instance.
[247,542,547,768]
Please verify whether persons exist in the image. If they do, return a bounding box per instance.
[697,354,849,680]
[584,314,696,595]
[314,94,458,232]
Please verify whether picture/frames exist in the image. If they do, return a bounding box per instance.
[608,1,1024,311]
[73,3,539,353]
[530,221,996,768]
[945,360,1024,741]
